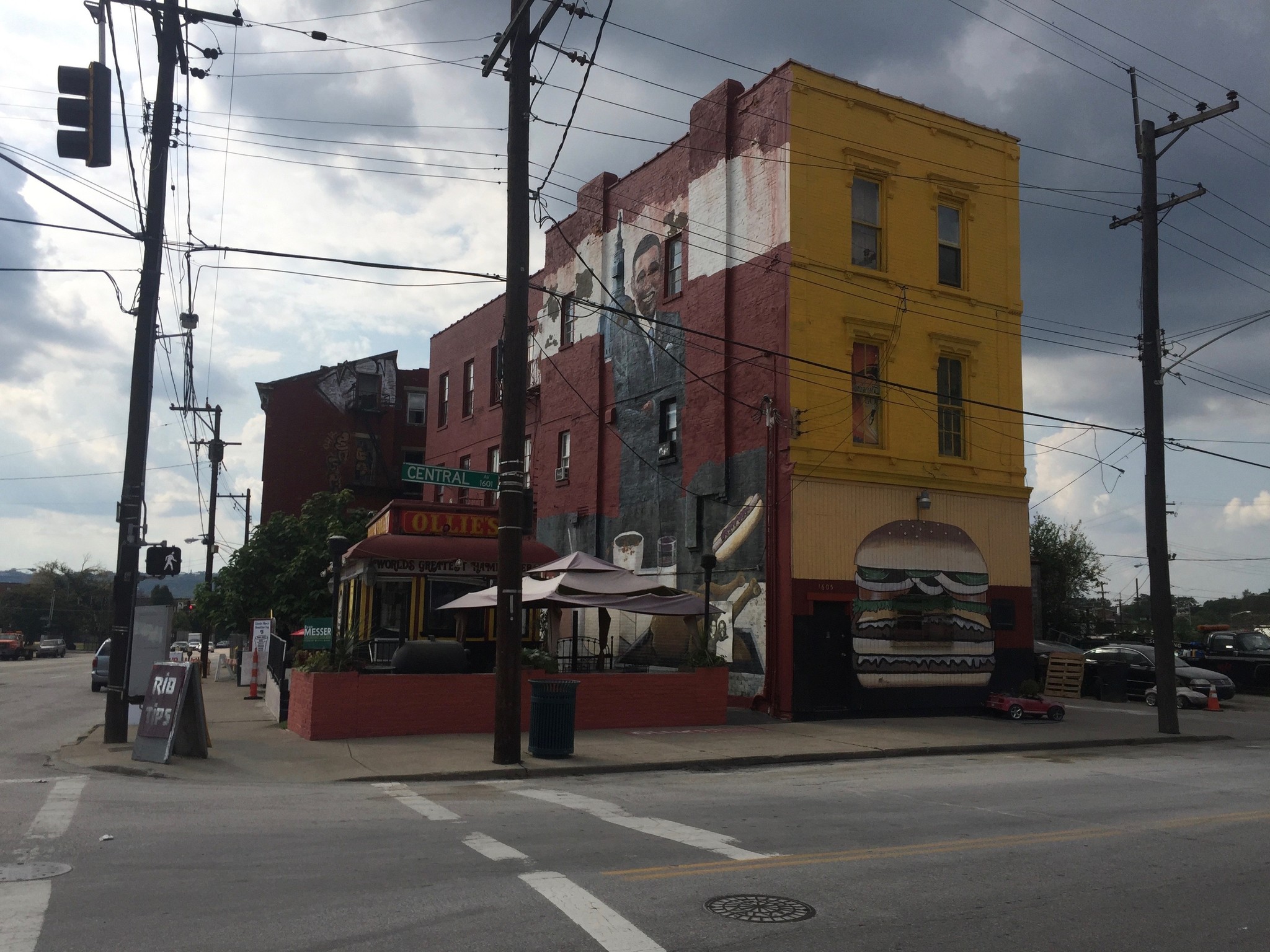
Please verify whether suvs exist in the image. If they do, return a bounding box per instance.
[39,638,66,658]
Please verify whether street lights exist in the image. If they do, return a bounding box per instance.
[183,537,249,688]
[1230,610,1251,630]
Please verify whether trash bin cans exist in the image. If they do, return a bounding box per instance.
[526,679,581,757]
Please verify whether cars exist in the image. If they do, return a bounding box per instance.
[984,691,1066,723]
[1081,643,1237,712]
[1144,684,1209,710]
[216,640,230,649]
[187,641,201,651]
[170,641,189,653]
[198,641,215,653]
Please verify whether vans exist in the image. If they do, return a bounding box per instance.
[90,637,109,692]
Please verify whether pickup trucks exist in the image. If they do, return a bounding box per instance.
[1174,628,1270,698]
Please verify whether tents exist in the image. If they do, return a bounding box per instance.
[435,551,725,675]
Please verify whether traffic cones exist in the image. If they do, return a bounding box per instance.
[1204,683,1225,712]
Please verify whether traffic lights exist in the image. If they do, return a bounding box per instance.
[56,59,112,168]
[146,546,181,576]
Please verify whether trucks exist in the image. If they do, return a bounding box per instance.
[0,630,38,661]
